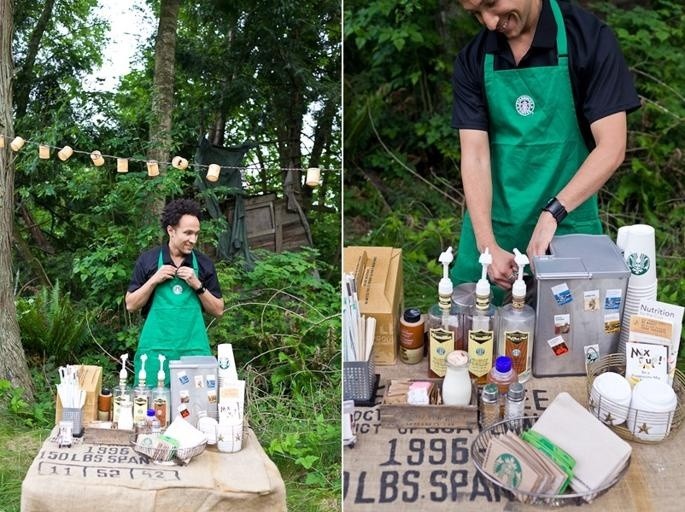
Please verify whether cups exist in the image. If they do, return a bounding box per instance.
[58,421,73,444]
[616,224,660,365]
[217,343,239,400]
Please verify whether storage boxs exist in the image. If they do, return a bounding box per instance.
[343,243,406,366]
[56,364,104,423]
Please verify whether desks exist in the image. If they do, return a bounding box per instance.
[343,348,685,511]
[17,423,289,512]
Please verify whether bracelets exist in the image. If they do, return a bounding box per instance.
[192,283,207,296]
[542,195,570,223]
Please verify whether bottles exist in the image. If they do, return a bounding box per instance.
[482,358,523,426]
[444,351,472,406]
[590,371,676,442]
[98,388,111,420]
[200,417,242,450]
[398,311,424,366]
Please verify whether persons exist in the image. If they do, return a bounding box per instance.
[124,198,224,387]
[441,1,643,316]
